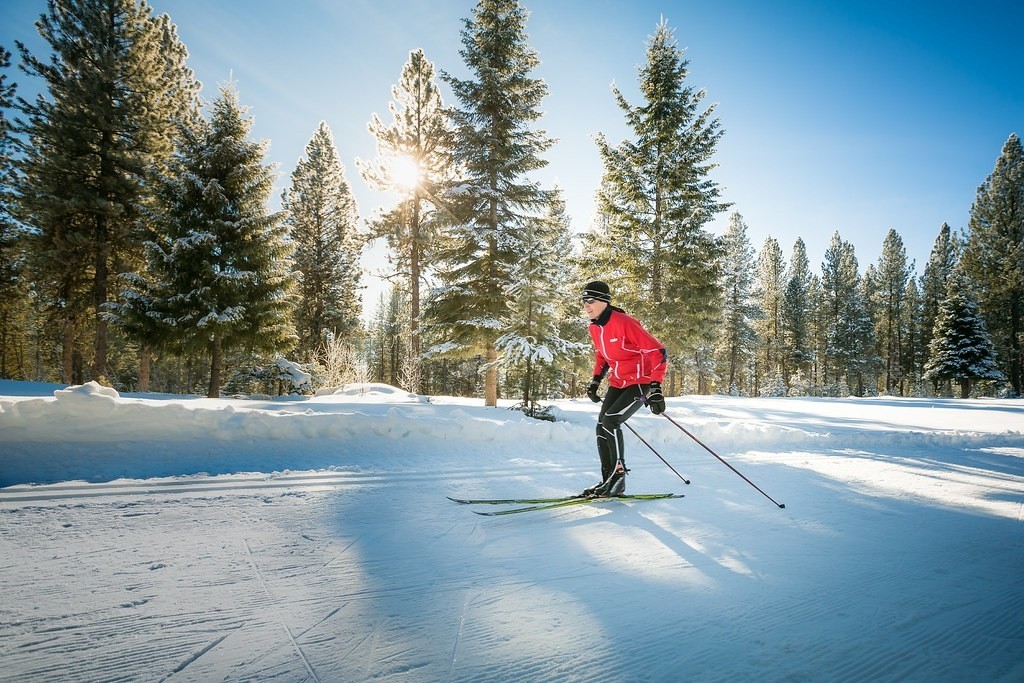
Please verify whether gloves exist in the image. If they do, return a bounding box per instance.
[587,376,601,403]
[644,382,665,415]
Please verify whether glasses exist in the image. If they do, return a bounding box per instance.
[583,298,595,304]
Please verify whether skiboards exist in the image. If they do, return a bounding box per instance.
[443,491,686,517]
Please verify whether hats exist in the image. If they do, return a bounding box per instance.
[582,281,611,302]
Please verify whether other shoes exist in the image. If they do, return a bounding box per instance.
[594,473,625,495]
[583,480,603,496]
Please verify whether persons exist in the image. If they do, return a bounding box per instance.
[582,282,667,496]
